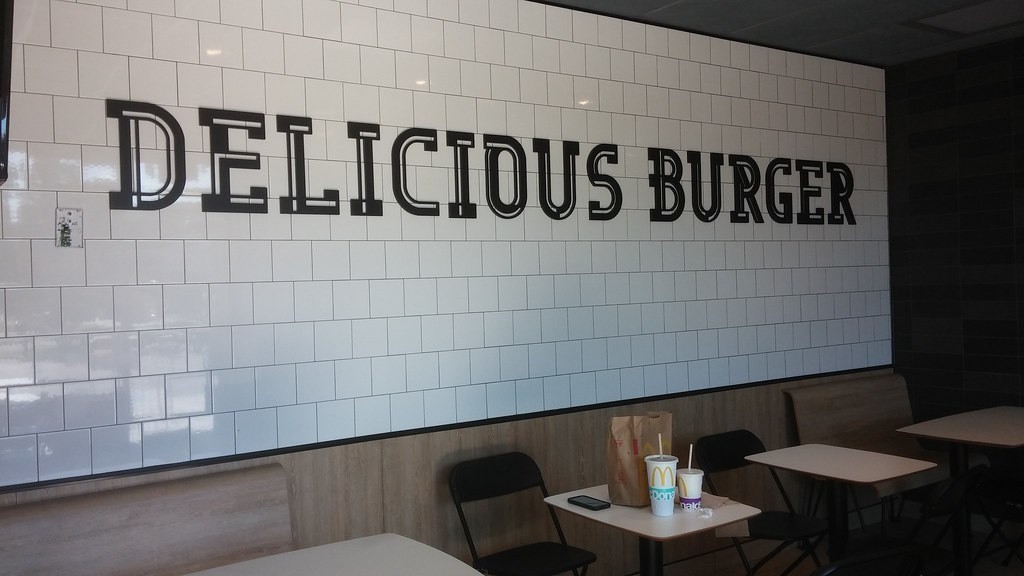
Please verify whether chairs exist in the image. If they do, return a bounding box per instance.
[447,451,599,575]
[782,372,997,541]
[0,462,290,576]
[691,430,841,576]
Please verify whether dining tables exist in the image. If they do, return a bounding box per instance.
[191,532,489,575]
[542,481,763,576]
[896,405,1023,574]
[745,443,937,573]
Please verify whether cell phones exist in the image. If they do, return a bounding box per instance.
[567,495,611,511]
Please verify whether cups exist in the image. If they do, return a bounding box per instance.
[644,454,679,516]
[676,467,705,512]
